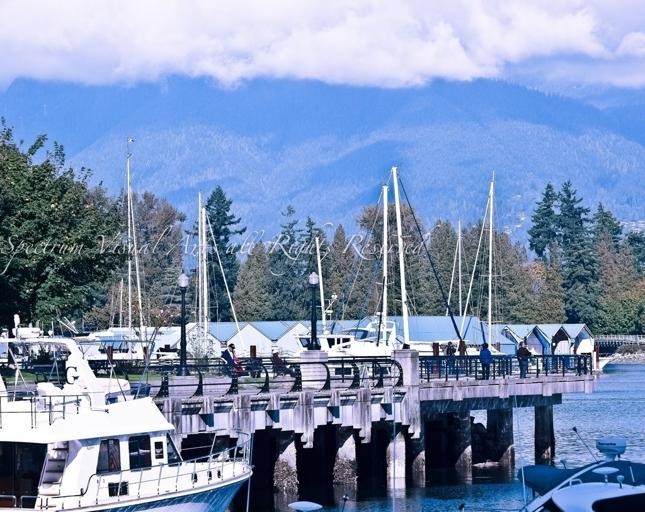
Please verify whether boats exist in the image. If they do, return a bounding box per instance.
[0,305,262,512]
[502,422,645,512]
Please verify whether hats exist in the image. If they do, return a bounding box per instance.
[273,349,279,354]
[229,344,235,349]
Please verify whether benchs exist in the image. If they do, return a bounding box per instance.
[30,363,66,388]
[268,357,302,376]
[217,356,263,377]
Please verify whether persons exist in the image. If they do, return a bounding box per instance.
[221,344,237,375]
[273,350,294,376]
[444,342,453,356]
[479,343,491,380]
[517,342,531,378]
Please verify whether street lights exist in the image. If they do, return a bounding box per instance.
[295,266,333,394]
[171,269,196,379]
